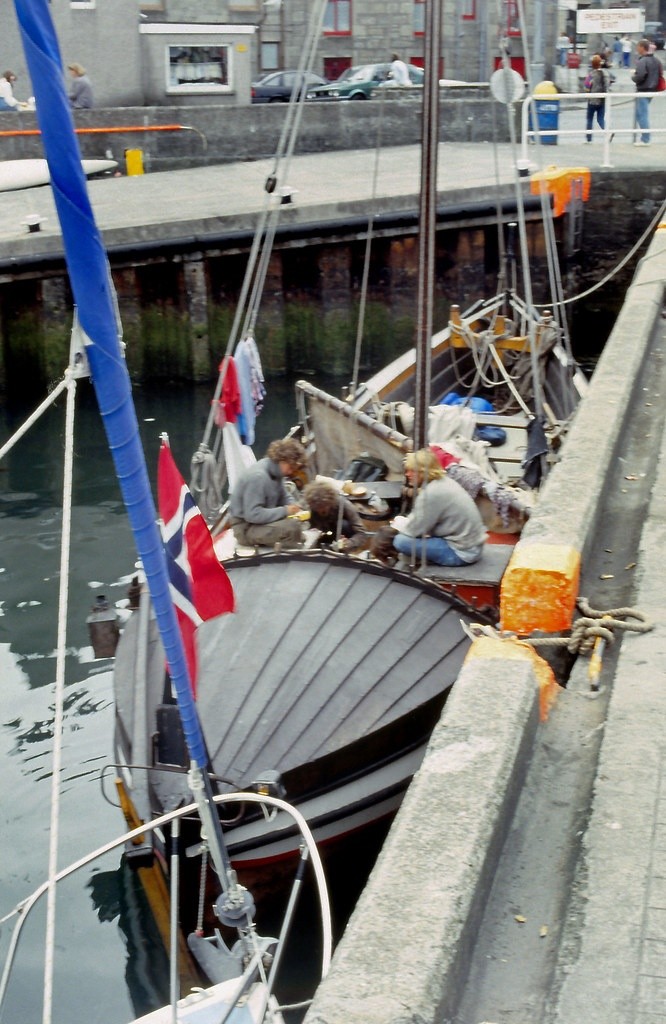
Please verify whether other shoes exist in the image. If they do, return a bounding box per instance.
[633,140,651,146]
[581,140,590,144]
[609,132,615,142]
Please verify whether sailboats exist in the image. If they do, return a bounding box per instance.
[110,0,591,926]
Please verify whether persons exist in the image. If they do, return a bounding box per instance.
[378,53,413,87]
[0,71,28,111]
[556,32,666,146]
[67,62,96,108]
[228,439,367,554]
[390,449,489,567]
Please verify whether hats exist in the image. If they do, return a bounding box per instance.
[592,54,601,70]
[67,63,84,77]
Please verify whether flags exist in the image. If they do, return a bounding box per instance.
[156,431,236,703]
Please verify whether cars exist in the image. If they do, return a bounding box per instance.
[305,62,427,102]
[251,70,330,104]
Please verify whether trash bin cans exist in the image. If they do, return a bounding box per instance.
[528,80,559,146]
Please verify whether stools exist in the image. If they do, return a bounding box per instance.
[418,544,514,609]
[212,528,322,561]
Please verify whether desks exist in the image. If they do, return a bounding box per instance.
[346,480,404,524]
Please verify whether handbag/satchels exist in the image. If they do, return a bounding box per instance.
[658,75,666,91]
